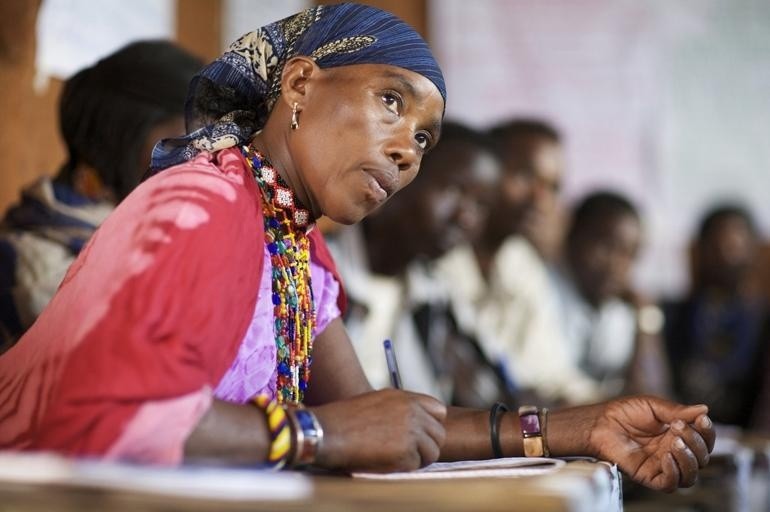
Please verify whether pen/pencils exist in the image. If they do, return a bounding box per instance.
[384,339,403,390]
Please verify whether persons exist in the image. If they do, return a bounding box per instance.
[1,38,205,353]
[3,2,718,493]
[317,117,770,510]
